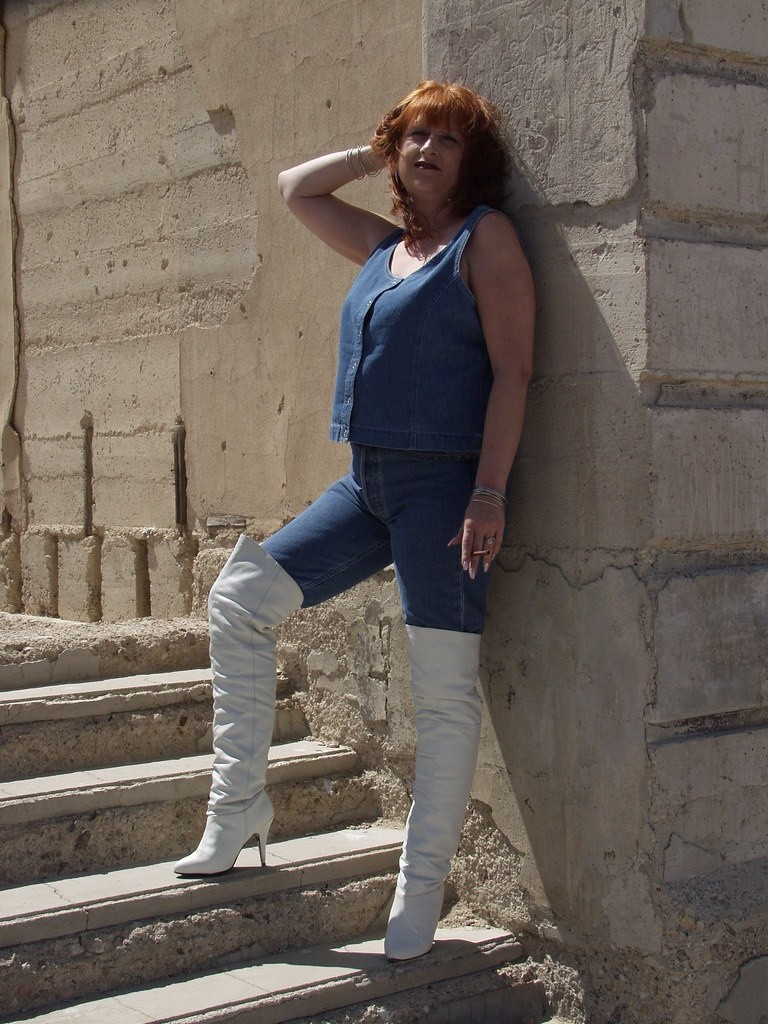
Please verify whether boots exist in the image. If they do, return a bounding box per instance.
[172,532,306,879]
[383,624,487,959]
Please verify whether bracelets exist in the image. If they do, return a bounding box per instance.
[470,485,508,511]
[346,145,382,181]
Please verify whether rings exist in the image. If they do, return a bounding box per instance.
[484,536,495,545]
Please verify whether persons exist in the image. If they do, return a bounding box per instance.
[172,81,540,962]
